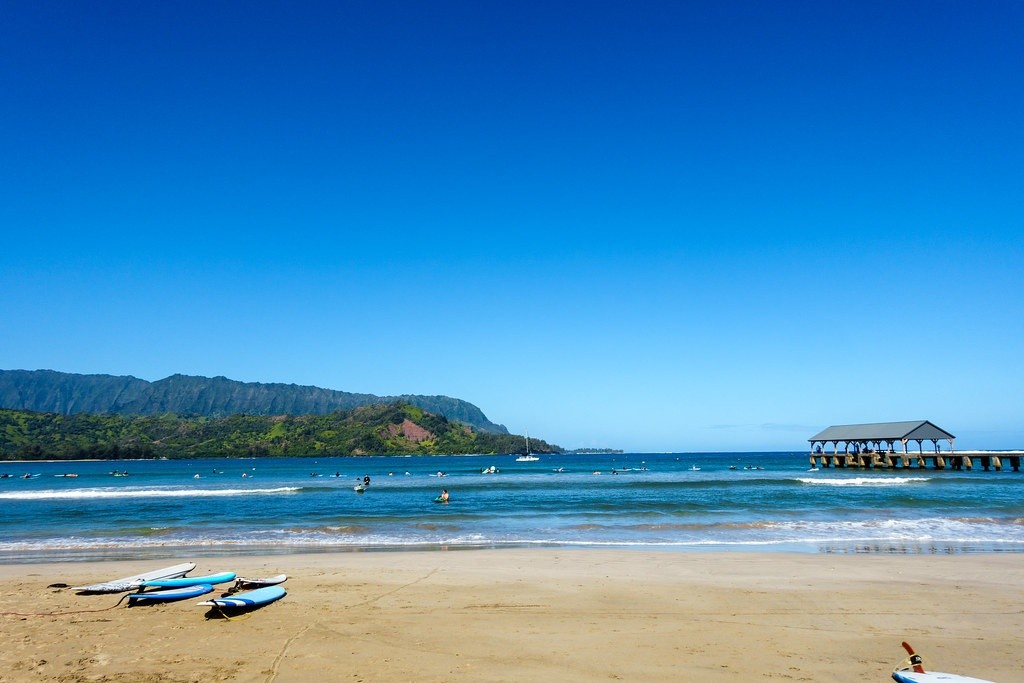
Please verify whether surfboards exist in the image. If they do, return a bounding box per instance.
[234,573,289,586]
[195,583,288,616]
[127,582,214,605]
[69,560,197,594]
[888,636,1022,683]
[139,567,236,590]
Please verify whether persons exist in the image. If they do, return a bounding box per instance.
[442,489,450,504]
[364,474,370,486]
[436,470,442,477]
[358,484,362,489]
[747,463,753,469]
[213,469,216,473]
[241,472,247,477]
[336,472,340,477]
[817,445,822,453]
[612,467,616,474]
[310,471,317,476]
